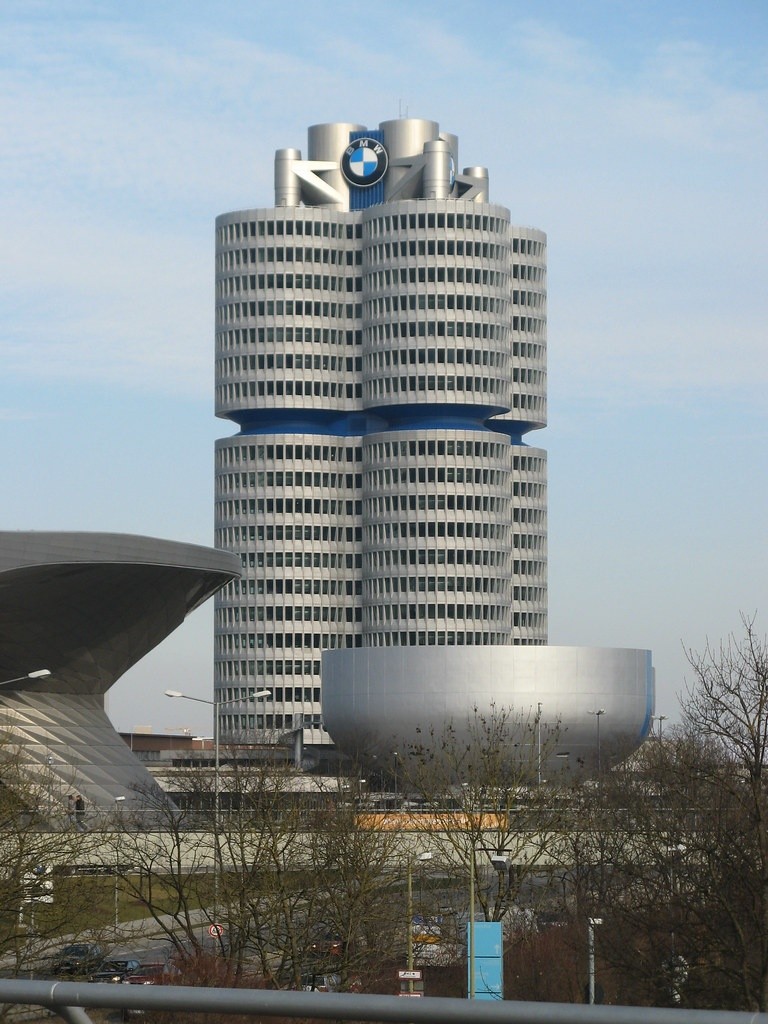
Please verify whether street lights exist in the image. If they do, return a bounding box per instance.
[164,690,271,973]
[470,847,512,999]
[408,853,432,992]
[358,779,366,803]
[651,715,669,797]
[588,709,606,773]
[587,917,603,1004]
[394,752,398,809]
[538,702,543,786]
[115,796,125,932]
[373,755,383,799]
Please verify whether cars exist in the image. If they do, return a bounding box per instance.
[89,960,140,984]
[122,963,180,985]
[301,973,340,993]
[52,944,104,975]
[310,932,361,960]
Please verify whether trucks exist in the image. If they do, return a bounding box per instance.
[412,912,463,967]
